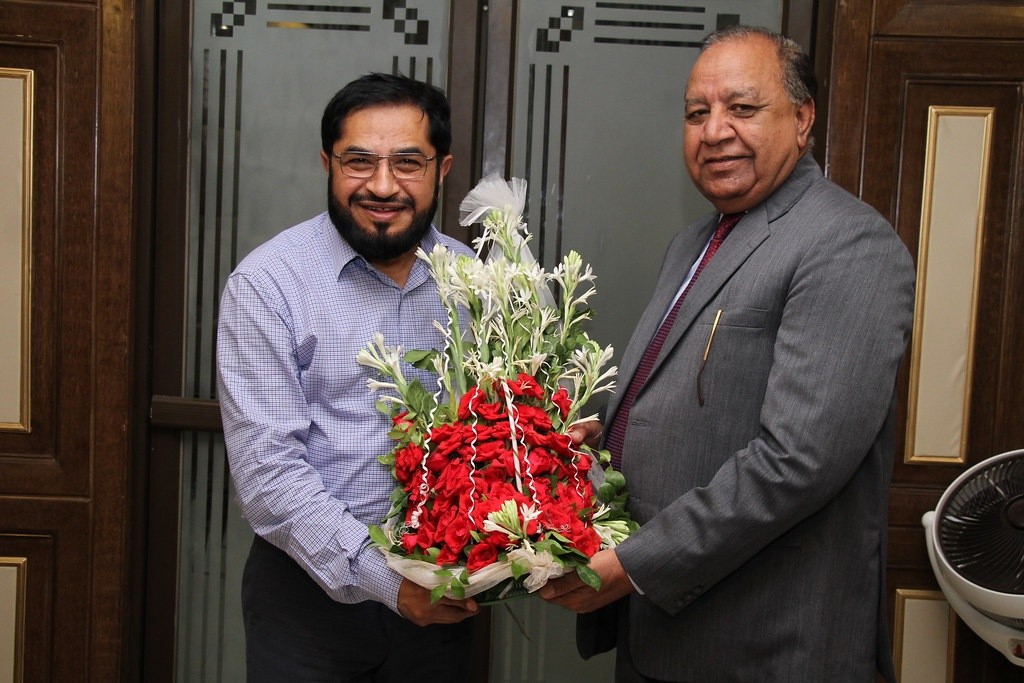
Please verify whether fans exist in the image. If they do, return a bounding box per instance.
[922,448,1024,667]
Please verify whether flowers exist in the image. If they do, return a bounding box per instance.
[357,170,643,608]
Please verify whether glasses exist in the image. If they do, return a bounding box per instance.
[332,151,437,179]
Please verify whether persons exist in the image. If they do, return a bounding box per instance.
[215,73,581,683]
[535,26,915,683]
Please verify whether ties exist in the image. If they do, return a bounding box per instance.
[601,212,746,496]
